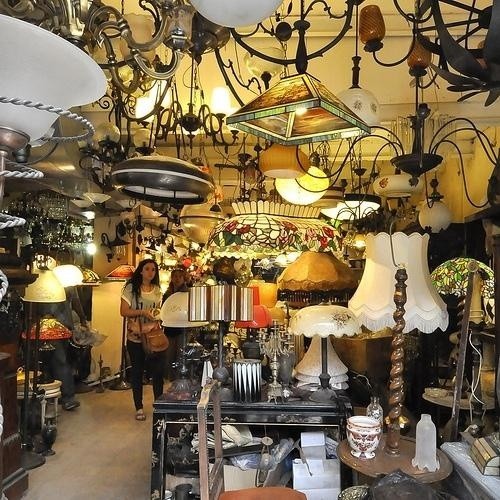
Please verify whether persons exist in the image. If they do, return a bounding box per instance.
[42,285,88,410]
[163,269,189,380]
[120,259,165,420]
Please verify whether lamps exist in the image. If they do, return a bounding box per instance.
[1,0,500,487]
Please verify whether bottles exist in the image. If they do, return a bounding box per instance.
[365,396,383,450]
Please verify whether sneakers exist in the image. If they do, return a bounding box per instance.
[61,401,80,410]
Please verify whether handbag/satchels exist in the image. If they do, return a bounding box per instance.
[140,322,170,356]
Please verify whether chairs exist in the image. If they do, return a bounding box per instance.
[196,377,307,500]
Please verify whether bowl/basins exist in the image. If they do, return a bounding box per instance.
[345,415,382,459]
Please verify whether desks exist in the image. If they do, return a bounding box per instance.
[336,431,453,486]
[150,375,355,500]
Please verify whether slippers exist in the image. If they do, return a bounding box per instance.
[135,412,147,421]
